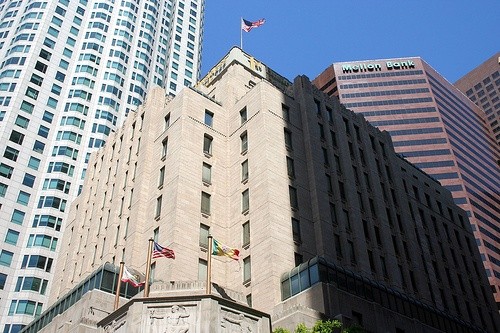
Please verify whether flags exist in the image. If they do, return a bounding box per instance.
[152,242,174,259]
[121,266,145,287]
[212,237,239,260]
[242,18,265,32]
